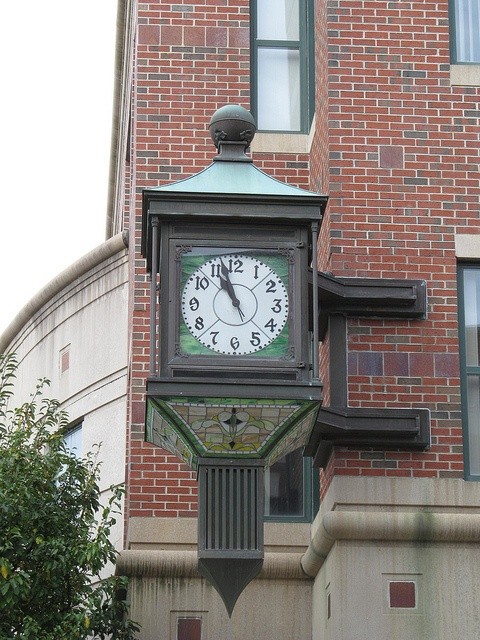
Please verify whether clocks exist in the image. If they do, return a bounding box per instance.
[166,235,304,370]
